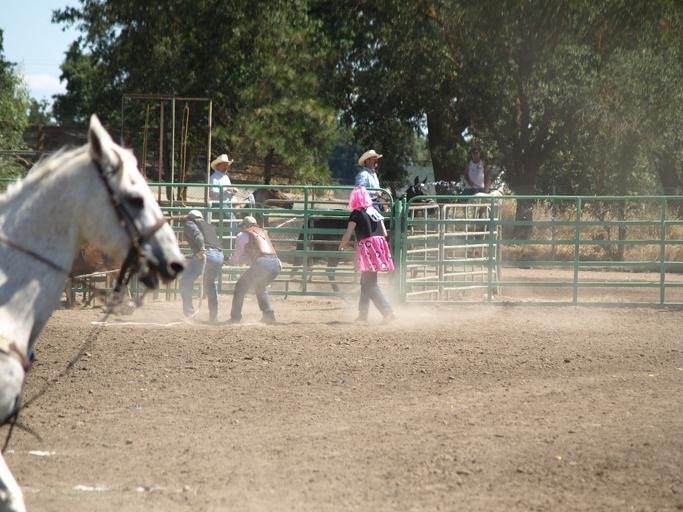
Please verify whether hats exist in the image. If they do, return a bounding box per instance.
[184,210,203,219]
[358,149,383,167]
[238,216,256,224]
[211,154,234,170]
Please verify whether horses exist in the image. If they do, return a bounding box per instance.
[291,175,438,292]
[211,183,293,248]
[0,111,188,512]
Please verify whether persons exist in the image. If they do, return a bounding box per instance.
[354,150,384,217]
[339,185,400,326]
[180,210,225,321]
[207,153,238,224]
[228,216,283,324]
[460,147,492,201]
